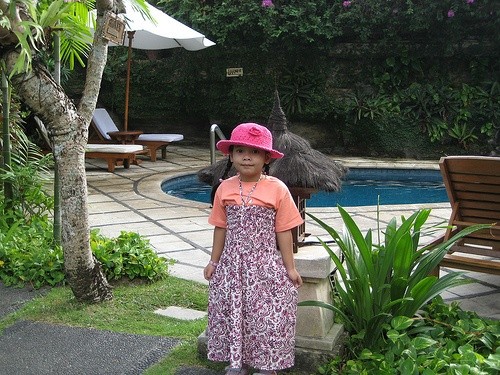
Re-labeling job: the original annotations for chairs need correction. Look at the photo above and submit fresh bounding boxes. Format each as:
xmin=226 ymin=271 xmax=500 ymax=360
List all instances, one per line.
xmin=418 ymin=156 xmax=500 ymax=279
xmin=34 ymin=115 xmax=143 ymax=171
xmin=91 ymin=109 xmax=184 ymax=159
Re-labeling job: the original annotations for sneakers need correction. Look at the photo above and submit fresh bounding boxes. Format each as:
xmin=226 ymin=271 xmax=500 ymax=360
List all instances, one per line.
xmin=224 ymin=366 xmax=248 ymax=375
xmin=253 ymin=370 xmax=277 ymax=375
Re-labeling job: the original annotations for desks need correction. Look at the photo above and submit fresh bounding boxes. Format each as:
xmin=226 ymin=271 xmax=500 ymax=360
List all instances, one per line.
xmin=105 ymin=131 xmax=145 ymax=165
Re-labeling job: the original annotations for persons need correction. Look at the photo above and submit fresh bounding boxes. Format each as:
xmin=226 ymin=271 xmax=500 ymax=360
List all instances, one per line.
xmin=204 ymin=123 xmax=304 ymax=375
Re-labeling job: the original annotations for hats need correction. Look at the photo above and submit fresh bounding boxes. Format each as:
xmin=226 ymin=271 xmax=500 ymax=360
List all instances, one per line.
xmin=216 ymin=122 xmax=284 ymax=158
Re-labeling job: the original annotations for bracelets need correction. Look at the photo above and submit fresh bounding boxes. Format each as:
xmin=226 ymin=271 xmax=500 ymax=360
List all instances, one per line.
xmin=210 ymin=260 xmax=218 ymax=266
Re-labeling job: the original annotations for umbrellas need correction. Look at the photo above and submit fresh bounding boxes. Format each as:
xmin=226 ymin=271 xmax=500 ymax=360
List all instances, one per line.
xmin=66 ymin=0 xmax=217 ymax=131
